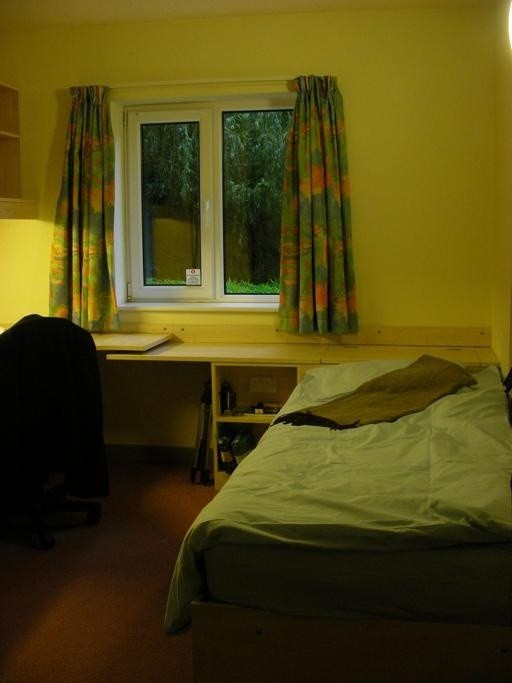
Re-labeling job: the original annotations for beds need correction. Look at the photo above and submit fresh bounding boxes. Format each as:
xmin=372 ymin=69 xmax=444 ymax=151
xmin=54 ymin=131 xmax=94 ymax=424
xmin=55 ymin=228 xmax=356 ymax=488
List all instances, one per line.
xmin=162 ymin=355 xmax=512 ymax=683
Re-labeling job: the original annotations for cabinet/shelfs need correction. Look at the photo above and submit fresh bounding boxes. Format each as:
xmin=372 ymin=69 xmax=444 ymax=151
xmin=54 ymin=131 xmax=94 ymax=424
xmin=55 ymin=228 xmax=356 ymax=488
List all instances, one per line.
xmin=0 ymin=82 xmax=41 ymax=219
xmin=210 ymin=362 xmax=301 ymax=493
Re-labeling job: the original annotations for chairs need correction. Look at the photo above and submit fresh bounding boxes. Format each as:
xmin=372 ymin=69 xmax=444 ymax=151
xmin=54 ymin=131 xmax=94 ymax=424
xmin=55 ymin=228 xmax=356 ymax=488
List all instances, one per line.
xmin=0 ymin=313 xmax=109 ymax=548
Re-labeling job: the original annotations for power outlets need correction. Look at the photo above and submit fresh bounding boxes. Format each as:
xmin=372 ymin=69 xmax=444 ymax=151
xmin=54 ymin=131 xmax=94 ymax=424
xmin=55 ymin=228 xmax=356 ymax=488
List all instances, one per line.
xmin=248 ymin=375 xmax=278 ymax=394
